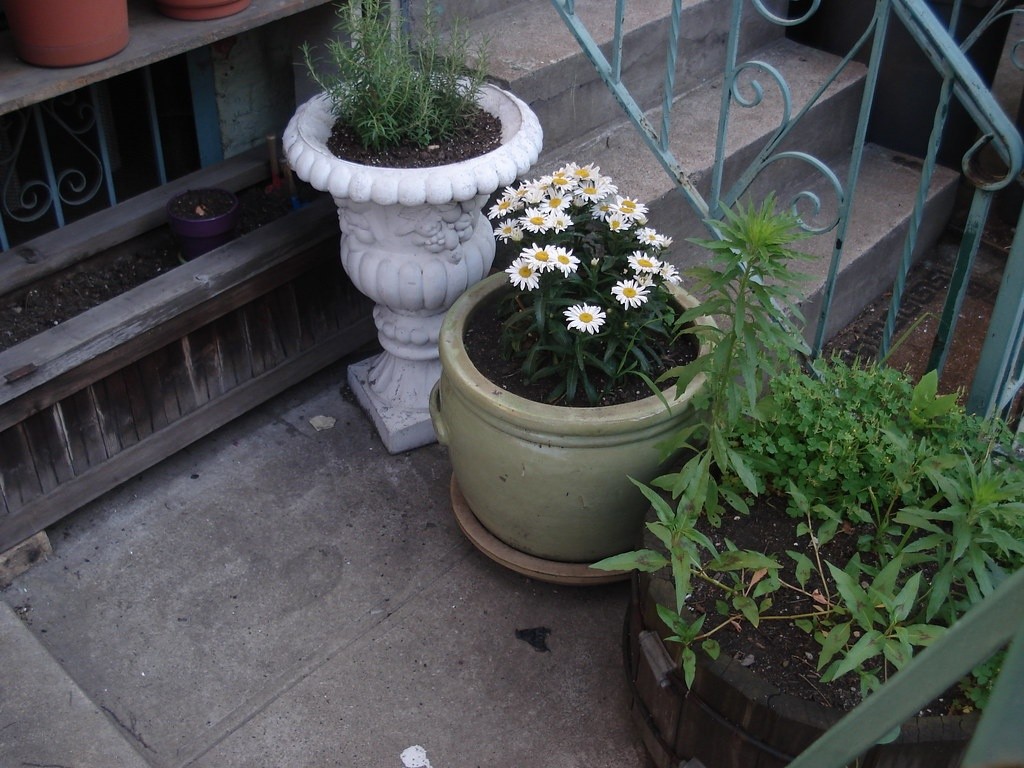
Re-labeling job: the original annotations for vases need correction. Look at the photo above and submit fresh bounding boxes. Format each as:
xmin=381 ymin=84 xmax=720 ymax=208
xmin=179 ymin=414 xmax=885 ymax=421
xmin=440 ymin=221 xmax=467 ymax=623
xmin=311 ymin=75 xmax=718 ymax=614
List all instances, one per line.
xmin=5 ymin=1 xmax=129 ymax=69
xmin=428 ymin=272 xmax=723 ymax=582
xmin=166 ymin=187 xmax=240 ymax=265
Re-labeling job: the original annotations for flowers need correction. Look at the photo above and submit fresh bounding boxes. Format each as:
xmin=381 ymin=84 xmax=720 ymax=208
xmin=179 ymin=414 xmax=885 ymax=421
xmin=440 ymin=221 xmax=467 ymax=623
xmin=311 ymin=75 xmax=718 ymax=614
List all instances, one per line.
xmin=484 ymin=162 xmax=676 ymax=404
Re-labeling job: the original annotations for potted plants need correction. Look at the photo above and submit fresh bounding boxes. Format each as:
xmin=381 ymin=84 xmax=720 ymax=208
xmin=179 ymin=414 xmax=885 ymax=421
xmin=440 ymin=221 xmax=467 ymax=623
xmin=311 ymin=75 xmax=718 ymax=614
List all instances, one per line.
xmin=622 ymin=191 xmax=1024 ymax=768
xmin=280 ymin=1 xmax=545 ymax=458
